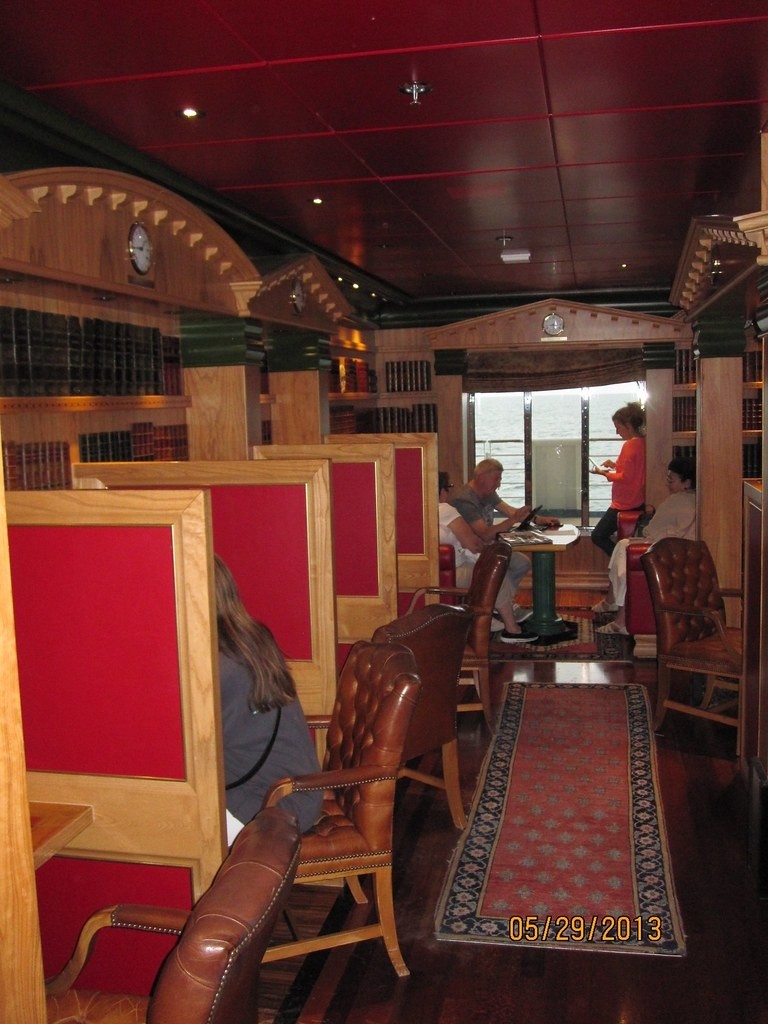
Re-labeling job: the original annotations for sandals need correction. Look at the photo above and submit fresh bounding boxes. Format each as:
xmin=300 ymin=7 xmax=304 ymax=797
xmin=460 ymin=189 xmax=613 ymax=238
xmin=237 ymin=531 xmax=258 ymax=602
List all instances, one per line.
xmin=590 ymin=599 xmax=618 ymax=612
xmin=594 ymin=621 xmax=630 ymax=636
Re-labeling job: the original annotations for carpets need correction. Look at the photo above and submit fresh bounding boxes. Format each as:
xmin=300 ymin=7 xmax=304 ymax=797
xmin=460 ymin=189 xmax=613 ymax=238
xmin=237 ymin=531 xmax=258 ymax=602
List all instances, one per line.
xmin=487 ymin=606 xmax=634 ymax=664
xmin=432 ymin=682 xmax=688 ymax=957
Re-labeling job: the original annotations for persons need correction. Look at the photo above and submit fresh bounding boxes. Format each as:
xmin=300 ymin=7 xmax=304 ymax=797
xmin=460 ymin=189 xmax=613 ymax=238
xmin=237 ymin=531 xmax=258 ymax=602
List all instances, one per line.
xmin=438 ymin=401 xmax=699 ymax=643
xmin=214 ymin=553 xmax=323 ymax=846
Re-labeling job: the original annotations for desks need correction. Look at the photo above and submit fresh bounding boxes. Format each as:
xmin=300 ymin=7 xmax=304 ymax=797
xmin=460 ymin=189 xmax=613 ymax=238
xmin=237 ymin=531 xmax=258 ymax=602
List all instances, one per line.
xmin=30 ymin=803 xmax=92 ymax=870
xmin=499 ymin=523 xmax=581 ymax=636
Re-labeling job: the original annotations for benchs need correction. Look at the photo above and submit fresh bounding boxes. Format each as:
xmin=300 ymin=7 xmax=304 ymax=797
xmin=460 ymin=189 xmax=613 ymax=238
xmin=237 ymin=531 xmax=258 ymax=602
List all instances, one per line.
xmin=617 ymin=509 xmax=658 ymax=660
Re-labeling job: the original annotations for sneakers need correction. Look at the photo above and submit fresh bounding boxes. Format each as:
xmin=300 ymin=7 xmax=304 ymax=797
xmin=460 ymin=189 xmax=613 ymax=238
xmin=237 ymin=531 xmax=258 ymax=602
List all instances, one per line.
xmin=492 ymin=603 xmax=533 ymax=623
xmin=500 ymin=626 xmax=539 ymax=644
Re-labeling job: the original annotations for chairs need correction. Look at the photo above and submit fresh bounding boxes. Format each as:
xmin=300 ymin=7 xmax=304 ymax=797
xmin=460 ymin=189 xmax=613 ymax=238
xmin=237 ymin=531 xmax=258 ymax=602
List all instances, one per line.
xmin=262 ymin=640 xmax=421 ymax=977
xmin=405 ymin=540 xmax=513 ymax=736
xmin=44 ymin=807 xmax=302 ymax=1024
xmin=640 ymin=536 xmax=741 ymax=759
xmin=371 ymin=603 xmax=475 ymax=830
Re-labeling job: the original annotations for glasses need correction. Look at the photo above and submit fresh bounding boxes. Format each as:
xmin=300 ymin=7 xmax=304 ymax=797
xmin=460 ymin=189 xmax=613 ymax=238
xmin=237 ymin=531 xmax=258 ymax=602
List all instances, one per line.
xmin=447 ymin=483 xmax=454 ymax=487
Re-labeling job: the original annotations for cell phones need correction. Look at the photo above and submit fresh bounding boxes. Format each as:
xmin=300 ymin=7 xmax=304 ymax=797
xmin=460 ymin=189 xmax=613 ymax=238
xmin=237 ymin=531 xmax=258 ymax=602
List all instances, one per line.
xmin=588 ymin=456 xmax=602 ymax=471
xmin=545 ymin=522 xmax=564 ymax=527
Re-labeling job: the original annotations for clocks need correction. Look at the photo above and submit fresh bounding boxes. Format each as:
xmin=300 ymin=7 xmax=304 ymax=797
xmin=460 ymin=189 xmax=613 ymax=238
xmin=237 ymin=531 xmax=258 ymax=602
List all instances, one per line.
xmin=542 ymin=313 xmax=565 ymax=336
xmin=290 ymin=277 xmax=304 ymax=313
xmin=128 ymin=222 xmax=153 ymax=275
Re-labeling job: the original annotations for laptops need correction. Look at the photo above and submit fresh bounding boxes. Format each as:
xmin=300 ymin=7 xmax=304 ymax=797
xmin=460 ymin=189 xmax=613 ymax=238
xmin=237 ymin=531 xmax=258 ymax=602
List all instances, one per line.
xmin=507 ymin=504 xmax=544 ymax=532
